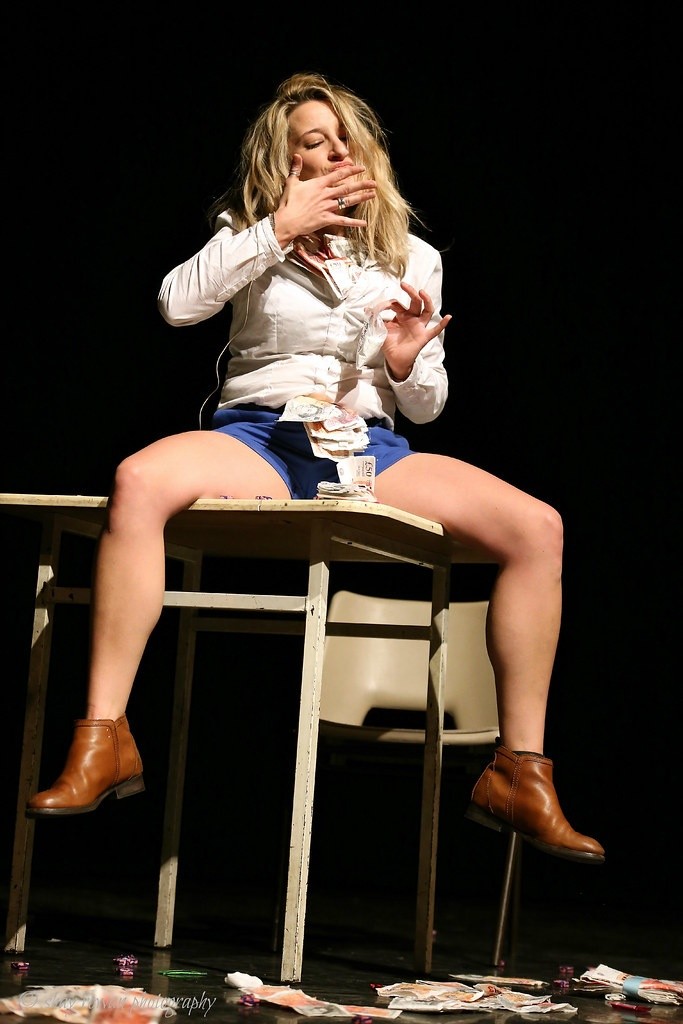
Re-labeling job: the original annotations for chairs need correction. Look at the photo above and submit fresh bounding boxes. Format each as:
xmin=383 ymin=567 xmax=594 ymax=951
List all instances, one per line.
xmin=272 ymin=591 xmax=529 ymax=975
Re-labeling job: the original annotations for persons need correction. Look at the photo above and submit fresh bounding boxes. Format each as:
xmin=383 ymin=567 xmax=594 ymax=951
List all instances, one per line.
xmin=26 ymin=76 xmax=606 ymax=863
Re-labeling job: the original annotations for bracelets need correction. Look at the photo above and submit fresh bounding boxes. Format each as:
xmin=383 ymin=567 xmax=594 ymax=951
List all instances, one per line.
xmin=269 ymin=211 xmax=276 ymax=235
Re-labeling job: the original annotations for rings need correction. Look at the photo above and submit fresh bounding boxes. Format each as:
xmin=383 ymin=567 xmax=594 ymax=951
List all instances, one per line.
xmin=337 ymin=198 xmax=346 ymax=210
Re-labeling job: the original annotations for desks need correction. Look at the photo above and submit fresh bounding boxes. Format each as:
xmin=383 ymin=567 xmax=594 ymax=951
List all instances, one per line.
xmin=0 ymin=494 xmax=456 ymax=984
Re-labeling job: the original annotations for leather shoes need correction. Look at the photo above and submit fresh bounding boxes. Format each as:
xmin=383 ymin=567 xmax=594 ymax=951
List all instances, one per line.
xmin=470 ymin=738 xmax=607 ymax=865
xmin=22 ymin=715 xmax=146 ymax=815
